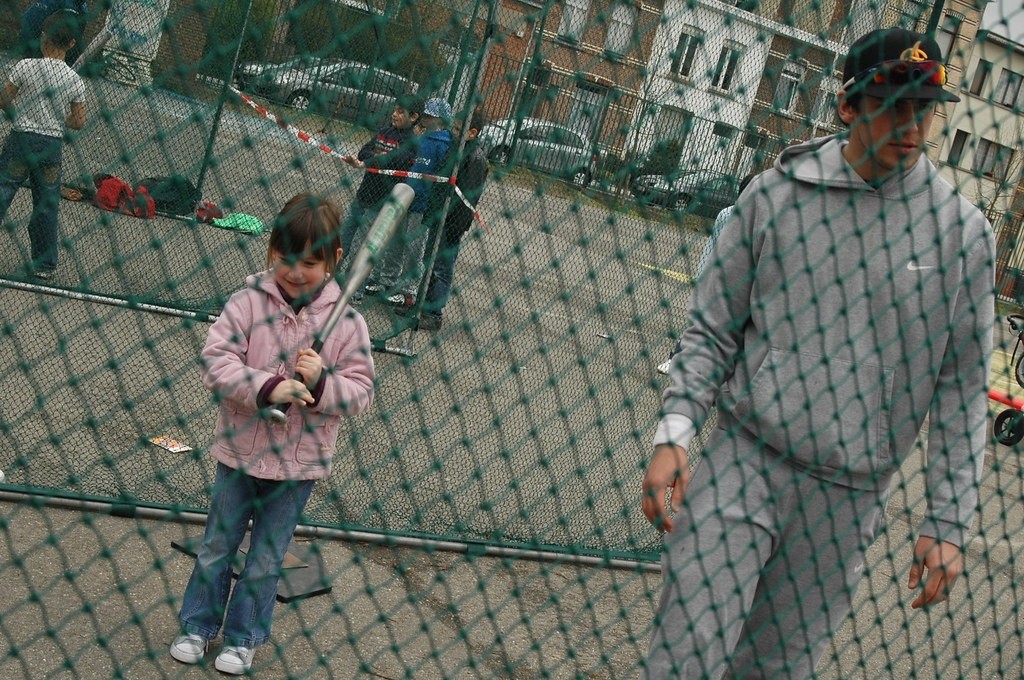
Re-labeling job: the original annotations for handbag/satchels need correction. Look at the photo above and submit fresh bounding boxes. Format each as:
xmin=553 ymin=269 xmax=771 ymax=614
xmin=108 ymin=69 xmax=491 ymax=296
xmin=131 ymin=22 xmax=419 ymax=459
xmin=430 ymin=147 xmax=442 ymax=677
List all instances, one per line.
xmin=92 ymin=173 xmax=155 ymax=218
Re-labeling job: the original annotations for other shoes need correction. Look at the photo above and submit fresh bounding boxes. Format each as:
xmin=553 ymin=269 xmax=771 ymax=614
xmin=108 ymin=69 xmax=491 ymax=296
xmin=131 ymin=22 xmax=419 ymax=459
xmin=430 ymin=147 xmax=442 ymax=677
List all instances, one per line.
xmin=405 ymin=294 xmax=417 ymax=304
xmin=33 ymin=267 xmax=56 ymax=278
xmin=365 ymin=281 xmax=392 ymax=295
xmin=394 ymin=305 xmax=416 ymax=316
xmin=419 ymin=313 xmax=442 ymax=330
xmin=384 ymin=292 xmax=407 ymax=305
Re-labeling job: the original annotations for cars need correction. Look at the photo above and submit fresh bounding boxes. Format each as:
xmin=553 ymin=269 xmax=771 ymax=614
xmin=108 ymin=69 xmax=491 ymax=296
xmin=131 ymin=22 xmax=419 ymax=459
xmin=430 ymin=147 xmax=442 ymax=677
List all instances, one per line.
xmin=476 ymin=116 xmax=598 ymax=188
xmin=629 ymin=169 xmax=742 ymax=218
xmin=236 ymin=55 xmax=322 ymax=87
xmin=251 ymin=58 xmax=418 ymax=132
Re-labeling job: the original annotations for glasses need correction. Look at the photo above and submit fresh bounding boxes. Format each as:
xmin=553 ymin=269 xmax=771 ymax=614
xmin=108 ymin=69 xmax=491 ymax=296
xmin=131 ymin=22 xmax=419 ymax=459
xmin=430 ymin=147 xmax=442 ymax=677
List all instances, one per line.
xmin=424 ymin=113 xmax=443 ymax=120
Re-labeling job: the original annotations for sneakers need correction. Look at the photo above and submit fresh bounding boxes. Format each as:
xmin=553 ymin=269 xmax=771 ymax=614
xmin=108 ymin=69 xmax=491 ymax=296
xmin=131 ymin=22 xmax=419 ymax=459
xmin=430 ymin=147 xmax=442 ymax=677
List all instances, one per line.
xmin=170 ymin=634 xmax=209 ymax=663
xmin=215 ymin=646 xmax=256 ymax=674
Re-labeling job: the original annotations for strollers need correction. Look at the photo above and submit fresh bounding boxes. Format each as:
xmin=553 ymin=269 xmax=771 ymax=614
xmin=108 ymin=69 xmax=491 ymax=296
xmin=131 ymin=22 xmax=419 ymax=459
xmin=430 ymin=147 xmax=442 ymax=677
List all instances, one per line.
xmin=994 ymin=313 xmax=1024 ymax=445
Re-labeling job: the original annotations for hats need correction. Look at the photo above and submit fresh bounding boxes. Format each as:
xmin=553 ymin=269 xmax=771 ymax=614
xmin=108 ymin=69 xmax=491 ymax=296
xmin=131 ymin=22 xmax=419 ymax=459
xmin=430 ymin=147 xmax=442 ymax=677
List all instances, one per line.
xmin=843 ymin=26 xmax=961 ymax=103
xmin=424 ymin=98 xmax=452 ymax=119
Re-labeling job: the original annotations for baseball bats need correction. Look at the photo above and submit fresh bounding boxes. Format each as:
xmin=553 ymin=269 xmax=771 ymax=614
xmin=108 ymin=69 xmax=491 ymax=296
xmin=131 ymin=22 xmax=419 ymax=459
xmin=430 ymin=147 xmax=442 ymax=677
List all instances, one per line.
xmin=269 ymin=182 xmax=415 ymax=423
xmin=70 ymin=26 xmax=114 ymax=73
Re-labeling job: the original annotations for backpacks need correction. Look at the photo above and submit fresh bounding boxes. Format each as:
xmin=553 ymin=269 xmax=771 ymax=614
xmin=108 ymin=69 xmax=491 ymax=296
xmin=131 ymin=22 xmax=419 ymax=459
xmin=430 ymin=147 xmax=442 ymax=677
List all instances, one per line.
xmin=134 ymin=176 xmax=196 ymax=215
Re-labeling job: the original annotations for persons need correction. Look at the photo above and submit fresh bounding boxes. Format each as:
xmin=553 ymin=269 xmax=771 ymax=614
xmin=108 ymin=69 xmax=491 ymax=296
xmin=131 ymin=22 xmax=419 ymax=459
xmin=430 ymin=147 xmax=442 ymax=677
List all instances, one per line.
xmin=20 ymin=0 xmax=90 ymax=62
xmin=335 ymin=93 xmax=490 ymax=332
xmin=166 ymin=193 xmax=376 ymax=679
xmin=640 ymin=26 xmax=1008 ymax=680
xmin=0 ymin=9 xmax=87 ymax=280
